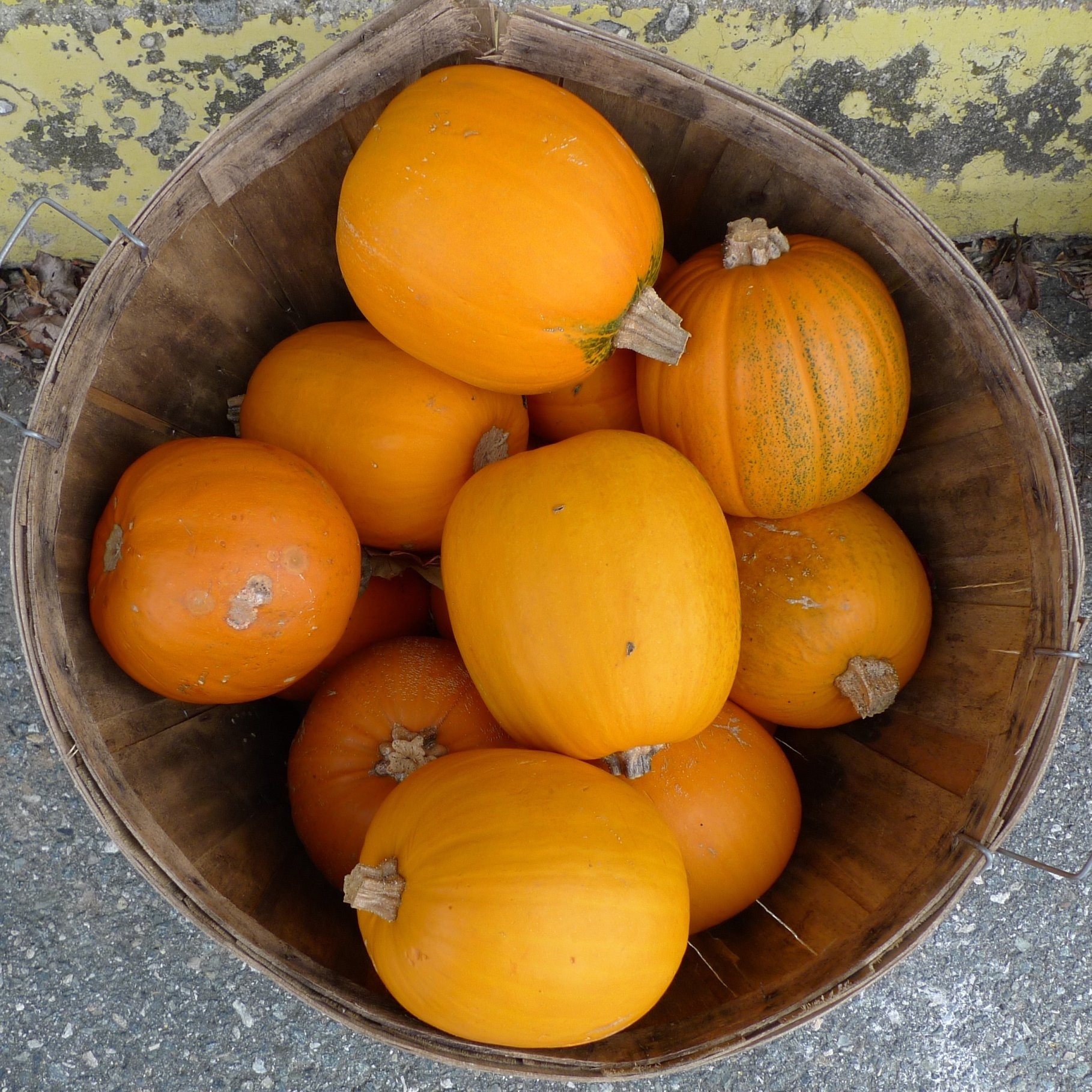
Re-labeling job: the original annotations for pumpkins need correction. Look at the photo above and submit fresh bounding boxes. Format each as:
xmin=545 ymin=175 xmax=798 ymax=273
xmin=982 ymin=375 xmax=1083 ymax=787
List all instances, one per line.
xmin=84 ymin=61 xmax=931 ymax=1052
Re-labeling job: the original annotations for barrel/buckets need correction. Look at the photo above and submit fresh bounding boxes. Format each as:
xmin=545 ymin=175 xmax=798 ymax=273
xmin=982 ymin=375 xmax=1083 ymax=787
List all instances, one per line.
xmin=1 ymin=1 xmax=1088 ymax=1084
